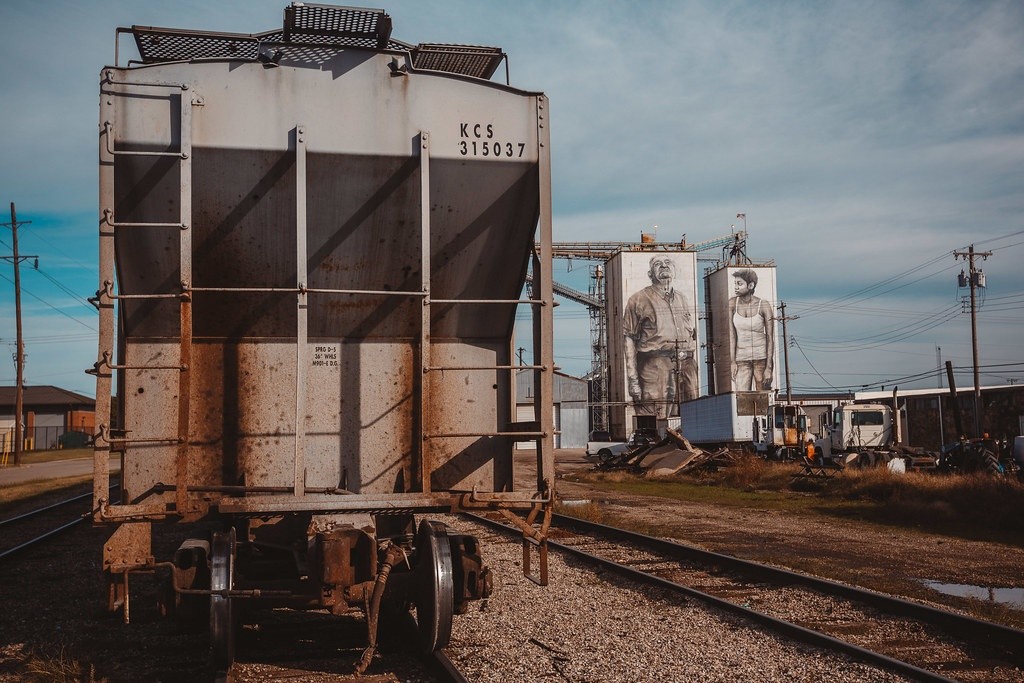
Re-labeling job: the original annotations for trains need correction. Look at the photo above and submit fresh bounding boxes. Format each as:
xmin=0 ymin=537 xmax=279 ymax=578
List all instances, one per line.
xmin=82 ymin=0 xmax=561 ymax=671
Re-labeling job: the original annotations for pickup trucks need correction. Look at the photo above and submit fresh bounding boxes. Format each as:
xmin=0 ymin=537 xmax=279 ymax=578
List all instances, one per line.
xmin=585 ymin=433 xmax=656 ymax=462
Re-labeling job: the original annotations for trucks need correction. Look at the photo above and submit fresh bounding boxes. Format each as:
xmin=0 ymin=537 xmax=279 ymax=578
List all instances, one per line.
xmin=814 ymin=385 xmax=939 ymax=473
